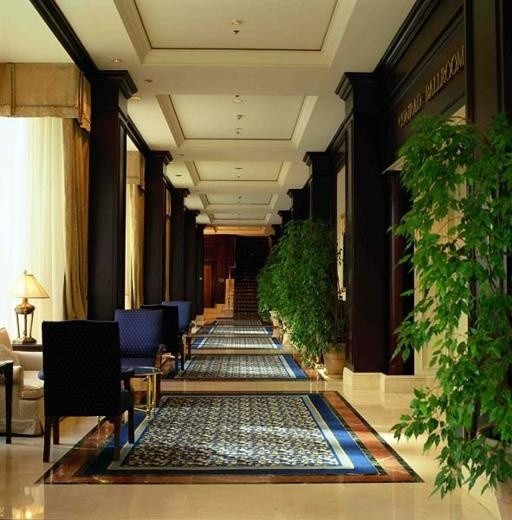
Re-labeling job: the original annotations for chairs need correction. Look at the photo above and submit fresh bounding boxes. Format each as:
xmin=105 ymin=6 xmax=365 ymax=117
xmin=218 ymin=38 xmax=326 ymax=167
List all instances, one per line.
xmin=0 ymin=327 xmax=61 ymax=435
xmin=41 ymin=300 xmax=192 ymax=461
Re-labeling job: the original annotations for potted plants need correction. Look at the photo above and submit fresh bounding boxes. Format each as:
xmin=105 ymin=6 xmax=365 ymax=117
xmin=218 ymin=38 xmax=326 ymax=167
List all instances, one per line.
xmin=394 ymin=115 xmax=512 ymax=520
xmin=258 ymin=216 xmax=349 ymax=377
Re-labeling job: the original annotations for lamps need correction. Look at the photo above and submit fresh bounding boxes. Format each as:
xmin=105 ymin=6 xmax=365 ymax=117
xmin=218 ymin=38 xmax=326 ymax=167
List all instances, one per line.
xmin=7 ymin=272 xmax=50 ymax=343
